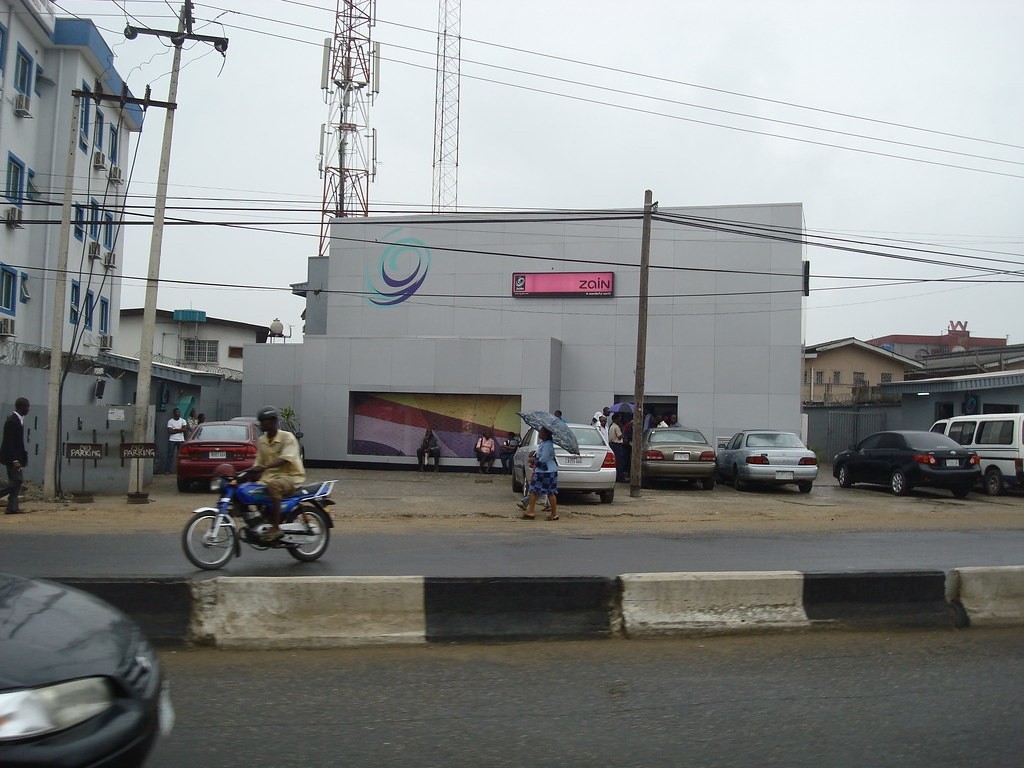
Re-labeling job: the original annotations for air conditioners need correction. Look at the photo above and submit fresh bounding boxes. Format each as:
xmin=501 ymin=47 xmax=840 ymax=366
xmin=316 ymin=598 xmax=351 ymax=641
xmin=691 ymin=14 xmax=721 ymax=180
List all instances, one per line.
xmin=8 ymin=206 xmax=23 ymax=229
xmin=99 ymin=334 xmax=113 ymax=350
xmin=110 ymin=166 xmax=121 ymax=182
xmin=0 ymin=317 xmax=16 ymax=337
xmin=88 ymin=242 xmax=101 ymax=259
xmin=94 ymin=151 xmax=106 ymax=170
xmin=16 ymin=93 xmax=31 ymax=117
xmin=103 ymin=252 xmax=116 ymax=268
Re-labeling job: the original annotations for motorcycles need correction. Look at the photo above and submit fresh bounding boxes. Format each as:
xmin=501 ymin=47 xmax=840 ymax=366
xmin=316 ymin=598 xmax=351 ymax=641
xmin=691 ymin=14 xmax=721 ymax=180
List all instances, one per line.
xmin=181 ymin=462 xmax=341 ymax=571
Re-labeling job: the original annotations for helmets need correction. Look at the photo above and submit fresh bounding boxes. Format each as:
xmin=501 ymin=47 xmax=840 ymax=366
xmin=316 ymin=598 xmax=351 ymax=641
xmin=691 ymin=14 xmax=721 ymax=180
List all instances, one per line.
xmin=211 ymin=464 xmax=234 ymax=482
xmin=258 ymin=405 xmax=278 ymax=421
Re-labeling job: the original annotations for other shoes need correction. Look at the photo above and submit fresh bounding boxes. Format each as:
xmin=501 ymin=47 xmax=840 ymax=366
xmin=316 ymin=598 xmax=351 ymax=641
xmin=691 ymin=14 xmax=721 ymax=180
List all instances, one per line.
xmin=517 ymin=503 xmax=527 ymax=510
xmin=434 ymin=469 xmax=438 ymax=472
xmin=541 ymin=508 xmax=552 ymax=511
xmin=262 ymin=530 xmax=284 ymax=541
xmin=618 ymin=478 xmax=630 ymax=483
xmin=166 ymin=471 xmax=170 ymax=475
xmin=4 ymin=508 xmax=23 ymax=514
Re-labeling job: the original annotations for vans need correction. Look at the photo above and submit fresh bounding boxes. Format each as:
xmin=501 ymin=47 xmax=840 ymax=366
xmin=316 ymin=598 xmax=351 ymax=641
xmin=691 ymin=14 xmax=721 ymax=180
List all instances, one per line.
xmin=929 ymin=413 xmax=1024 ymax=497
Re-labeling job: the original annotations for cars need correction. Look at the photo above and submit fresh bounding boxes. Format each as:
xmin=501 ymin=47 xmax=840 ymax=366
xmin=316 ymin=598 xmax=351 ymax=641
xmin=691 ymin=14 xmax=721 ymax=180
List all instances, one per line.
xmin=176 ymin=419 xmax=266 ymax=494
xmin=509 ymin=422 xmax=617 ymax=504
xmin=230 ymin=416 xmax=305 ymax=466
xmin=719 ymin=429 xmax=818 ymax=493
xmin=629 ymin=426 xmax=718 ymax=491
xmin=0 ymin=570 xmax=177 ymax=768
xmin=832 ymin=429 xmax=982 ymax=499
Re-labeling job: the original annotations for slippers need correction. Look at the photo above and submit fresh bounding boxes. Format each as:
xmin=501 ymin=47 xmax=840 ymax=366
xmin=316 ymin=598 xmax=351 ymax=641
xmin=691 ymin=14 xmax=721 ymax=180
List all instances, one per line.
xmin=545 ymin=514 xmax=559 ymax=521
xmin=522 ymin=514 xmax=535 ymax=520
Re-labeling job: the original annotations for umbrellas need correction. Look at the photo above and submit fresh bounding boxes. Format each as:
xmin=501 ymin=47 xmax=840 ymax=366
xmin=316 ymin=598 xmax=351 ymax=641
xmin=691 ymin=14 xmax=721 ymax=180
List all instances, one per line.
xmin=515 ymin=411 xmax=580 ymax=458
xmin=607 ymin=402 xmax=634 ymax=418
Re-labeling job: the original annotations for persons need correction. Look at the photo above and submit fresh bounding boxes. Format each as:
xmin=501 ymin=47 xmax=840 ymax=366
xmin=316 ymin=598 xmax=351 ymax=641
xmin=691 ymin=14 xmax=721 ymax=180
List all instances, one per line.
xmin=475 ymin=431 xmax=496 ymax=474
xmin=500 ymin=432 xmax=519 ymax=475
xmin=185 ymin=408 xmax=199 ymax=441
xmin=0 ymin=397 xmax=30 ymax=514
xmin=591 ymin=407 xmax=681 ymax=483
xmin=416 ymin=427 xmax=441 ymax=472
xmin=516 ymin=427 xmax=559 ymax=521
xmin=555 ymin=410 xmax=568 ymax=423
xmin=235 ymin=405 xmax=307 ymax=542
xmin=163 ymin=408 xmax=188 ymax=474
xmin=198 ymin=414 xmax=205 ymax=424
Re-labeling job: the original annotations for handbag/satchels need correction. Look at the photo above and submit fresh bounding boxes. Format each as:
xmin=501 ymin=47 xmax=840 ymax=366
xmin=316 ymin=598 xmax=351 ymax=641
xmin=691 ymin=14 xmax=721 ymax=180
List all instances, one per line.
xmin=425 ymin=453 xmax=428 ymax=465
xmin=474 ymin=437 xmax=483 ymax=453
